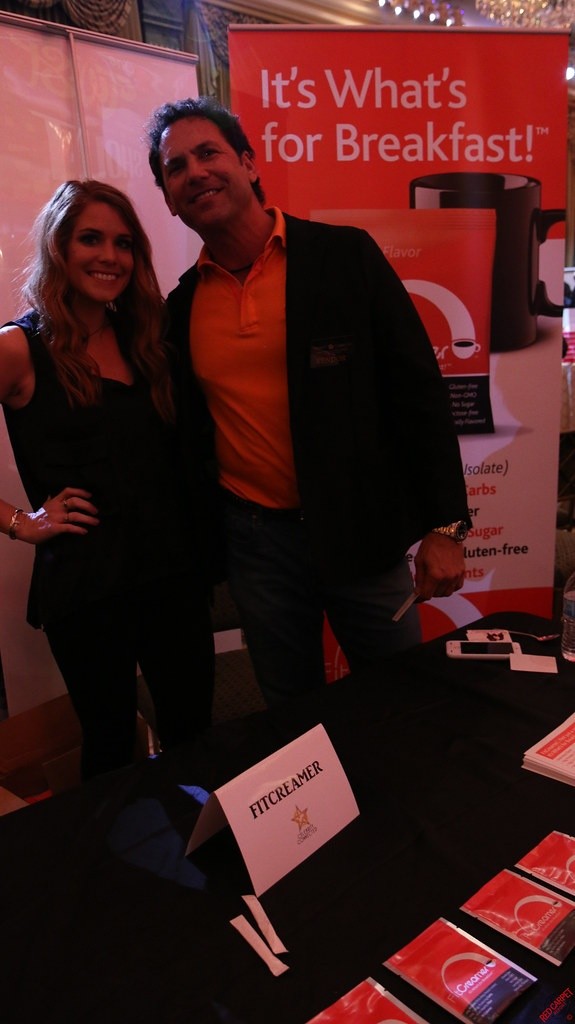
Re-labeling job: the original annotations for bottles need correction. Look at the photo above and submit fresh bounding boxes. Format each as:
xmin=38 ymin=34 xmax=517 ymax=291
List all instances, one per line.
xmin=561 ymin=572 xmax=575 ymax=661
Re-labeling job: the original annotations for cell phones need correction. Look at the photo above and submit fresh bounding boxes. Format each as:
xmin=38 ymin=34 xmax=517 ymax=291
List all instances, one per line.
xmin=445 ymin=641 xmax=522 ymax=659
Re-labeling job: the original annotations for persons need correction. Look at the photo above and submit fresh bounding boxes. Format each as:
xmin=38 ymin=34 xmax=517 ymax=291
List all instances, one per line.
xmin=148 ymin=99 xmax=472 ymax=710
xmin=0 ymin=181 xmax=224 ymax=782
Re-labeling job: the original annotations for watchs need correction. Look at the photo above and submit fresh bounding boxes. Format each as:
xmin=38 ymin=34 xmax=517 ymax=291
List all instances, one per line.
xmin=432 ymin=521 xmax=469 ymax=543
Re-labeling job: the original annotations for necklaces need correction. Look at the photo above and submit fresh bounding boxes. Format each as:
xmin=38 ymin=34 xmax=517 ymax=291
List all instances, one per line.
xmin=228 ymin=262 xmax=253 ymax=273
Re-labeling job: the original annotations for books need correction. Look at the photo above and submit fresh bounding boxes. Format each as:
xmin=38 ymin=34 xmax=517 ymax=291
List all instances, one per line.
xmin=520 ymin=711 xmax=575 ymax=787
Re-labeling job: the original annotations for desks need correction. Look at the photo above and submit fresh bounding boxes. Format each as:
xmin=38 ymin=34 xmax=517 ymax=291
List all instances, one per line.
xmin=0 ymin=610 xmax=575 ymax=1024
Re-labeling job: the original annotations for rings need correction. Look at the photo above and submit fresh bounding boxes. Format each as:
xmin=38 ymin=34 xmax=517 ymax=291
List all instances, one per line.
xmin=63 ymin=499 xmax=68 ymax=508
xmin=64 ymin=512 xmax=69 ymax=522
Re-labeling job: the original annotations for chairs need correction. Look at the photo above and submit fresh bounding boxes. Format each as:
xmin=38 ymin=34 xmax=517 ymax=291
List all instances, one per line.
xmin=130 ymin=581 xmax=268 ymax=738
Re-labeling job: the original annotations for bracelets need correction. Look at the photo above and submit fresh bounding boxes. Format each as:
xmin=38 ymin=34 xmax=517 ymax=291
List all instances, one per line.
xmin=9 ymin=508 xmax=24 ymax=540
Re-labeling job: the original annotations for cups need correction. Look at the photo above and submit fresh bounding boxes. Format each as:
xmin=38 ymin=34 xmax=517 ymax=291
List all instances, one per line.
xmin=452 ymin=340 xmax=480 ymax=359
xmin=410 ymin=173 xmax=566 ymax=352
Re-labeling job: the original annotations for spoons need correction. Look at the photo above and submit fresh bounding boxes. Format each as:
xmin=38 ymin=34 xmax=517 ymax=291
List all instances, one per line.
xmin=493 ymin=629 xmax=559 ymax=640
